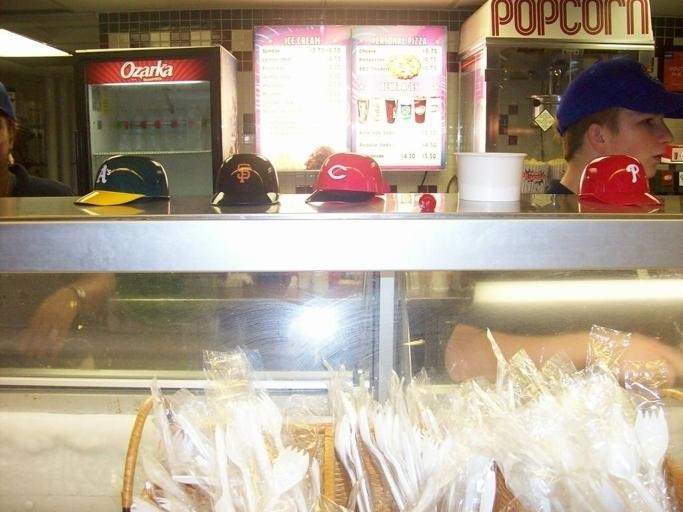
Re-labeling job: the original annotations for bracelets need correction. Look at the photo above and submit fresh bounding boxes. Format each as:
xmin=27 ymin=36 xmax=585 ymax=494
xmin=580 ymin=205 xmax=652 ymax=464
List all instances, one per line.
xmin=68 ymin=283 xmax=90 ymax=308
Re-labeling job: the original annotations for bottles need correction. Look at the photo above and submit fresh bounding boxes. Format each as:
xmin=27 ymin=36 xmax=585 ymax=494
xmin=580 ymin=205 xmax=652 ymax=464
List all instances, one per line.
xmin=356 ymin=95 xmax=441 ymax=124
xmin=115 ymin=102 xmax=212 ymax=152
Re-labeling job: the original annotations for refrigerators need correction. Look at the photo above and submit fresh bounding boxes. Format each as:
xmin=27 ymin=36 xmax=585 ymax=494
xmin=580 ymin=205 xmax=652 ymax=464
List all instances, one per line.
xmin=74 ymin=45 xmax=244 ymax=198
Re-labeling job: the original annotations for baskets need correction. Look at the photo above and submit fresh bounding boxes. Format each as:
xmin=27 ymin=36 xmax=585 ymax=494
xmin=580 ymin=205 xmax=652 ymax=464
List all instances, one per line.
xmin=122 ymin=387 xmax=682 ymax=512
xmin=120 ymin=387 xmax=682 ymax=512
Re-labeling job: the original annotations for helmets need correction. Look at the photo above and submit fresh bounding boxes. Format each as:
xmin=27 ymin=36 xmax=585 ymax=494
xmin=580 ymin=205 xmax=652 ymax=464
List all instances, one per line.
xmin=210 ymin=154 xmax=280 ymax=207
xmin=579 ymin=154 xmax=663 ymax=206
xmin=305 ymin=153 xmax=384 ymax=204
xmin=73 ymin=153 xmax=172 ymax=205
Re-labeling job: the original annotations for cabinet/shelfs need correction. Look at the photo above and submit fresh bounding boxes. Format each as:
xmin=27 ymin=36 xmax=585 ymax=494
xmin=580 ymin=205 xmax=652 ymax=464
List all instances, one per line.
xmin=0 ymin=192 xmax=683 ymax=512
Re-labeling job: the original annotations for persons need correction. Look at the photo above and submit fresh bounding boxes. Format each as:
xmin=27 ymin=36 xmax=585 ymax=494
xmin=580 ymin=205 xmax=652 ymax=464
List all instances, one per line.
xmin=0 ymin=78 xmax=118 ymax=366
xmin=422 ymin=54 xmax=683 ymax=392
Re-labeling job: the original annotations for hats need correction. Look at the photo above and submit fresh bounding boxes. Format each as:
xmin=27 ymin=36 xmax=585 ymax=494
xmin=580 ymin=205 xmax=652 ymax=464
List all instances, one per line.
xmin=556 ymin=60 xmax=683 ymax=134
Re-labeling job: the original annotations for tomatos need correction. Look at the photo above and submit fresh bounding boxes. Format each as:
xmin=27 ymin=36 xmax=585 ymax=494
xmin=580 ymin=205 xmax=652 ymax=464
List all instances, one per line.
xmin=419 ymin=194 xmax=436 ymax=212
